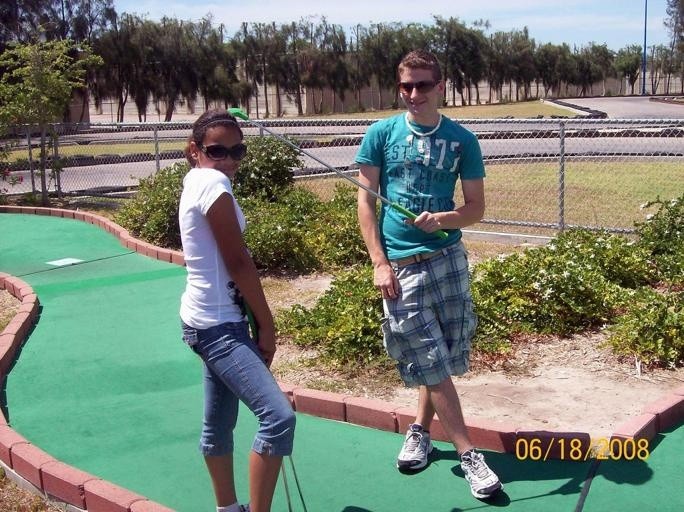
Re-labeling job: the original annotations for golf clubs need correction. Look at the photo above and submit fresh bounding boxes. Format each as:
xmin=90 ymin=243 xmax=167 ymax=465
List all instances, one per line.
xmin=242 ymin=304 xmax=307 ymax=512
xmin=227 ymin=107 xmax=448 ymax=240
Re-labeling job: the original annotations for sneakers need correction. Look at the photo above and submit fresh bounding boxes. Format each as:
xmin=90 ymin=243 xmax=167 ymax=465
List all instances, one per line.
xmin=460 ymin=449 xmax=504 ymax=499
xmin=397 ymin=423 xmax=433 ymax=469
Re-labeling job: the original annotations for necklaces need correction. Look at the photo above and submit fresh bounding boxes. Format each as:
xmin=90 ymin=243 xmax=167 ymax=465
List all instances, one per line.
xmin=403 ymin=109 xmax=442 ymax=139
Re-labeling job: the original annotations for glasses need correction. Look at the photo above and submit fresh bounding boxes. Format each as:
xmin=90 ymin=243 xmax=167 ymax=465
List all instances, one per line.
xmin=197 ymin=143 xmax=248 ymax=161
xmin=398 ymin=80 xmax=440 ymax=93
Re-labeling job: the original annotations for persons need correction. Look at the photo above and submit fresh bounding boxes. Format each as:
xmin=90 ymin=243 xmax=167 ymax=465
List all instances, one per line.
xmin=354 ymin=51 xmax=504 ymax=502
xmin=177 ymin=107 xmax=297 ymax=512
